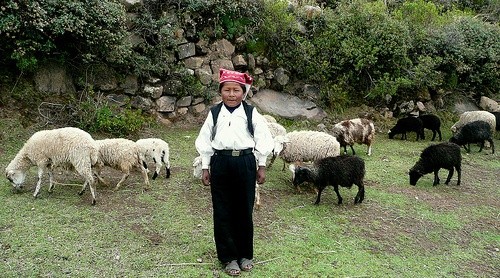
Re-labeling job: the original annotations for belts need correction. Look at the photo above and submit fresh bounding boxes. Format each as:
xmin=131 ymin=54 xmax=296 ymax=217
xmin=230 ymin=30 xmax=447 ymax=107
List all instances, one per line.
xmin=217 ymin=149 xmax=254 ymax=157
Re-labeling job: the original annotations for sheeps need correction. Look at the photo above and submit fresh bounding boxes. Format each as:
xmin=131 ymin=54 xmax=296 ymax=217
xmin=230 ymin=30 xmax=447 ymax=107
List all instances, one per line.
xmin=409 ymin=141 xmax=462 ymax=187
xmin=95 ymin=138 xmax=150 ymax=191
xmin=449 ymin=120 xmax=495 ymax=154
xmin=136 ymin=138 xmax=170 ymax=180
xmin=273 ymin=130 xmax=340 ymax=163
xmin=252 ymin=181 xmax=260 ymax=212
xmin=388 ymin=114 xmax=442 ymax=141
xmin=293 ymin=153 xmax=365 ymax=206
xmin=192 ymin=156 xmax=211 ymax=186
xmin=4 ymin=127 xmax=98 ymax=205
xmin=266 ymin=123 xmax=287 ymax=171
xmin=331 ymin=118 xmax=375 ymax=156
xmin=262 ymin=115 xmax=277 ymax=124
xmin=451 ymin=111 xmax=496 ymax=137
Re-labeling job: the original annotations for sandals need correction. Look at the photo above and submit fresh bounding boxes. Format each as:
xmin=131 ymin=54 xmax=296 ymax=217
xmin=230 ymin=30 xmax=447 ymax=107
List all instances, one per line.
xmin=225 ymin=260 xmax=242 ymax=276
xmin=240 ymin=257 xmax=255 ymax=272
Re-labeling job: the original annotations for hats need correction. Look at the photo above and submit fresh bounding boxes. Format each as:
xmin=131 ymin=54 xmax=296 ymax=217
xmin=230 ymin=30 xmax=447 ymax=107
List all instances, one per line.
xmin=218 ymin=67 xmax=254 ymax=102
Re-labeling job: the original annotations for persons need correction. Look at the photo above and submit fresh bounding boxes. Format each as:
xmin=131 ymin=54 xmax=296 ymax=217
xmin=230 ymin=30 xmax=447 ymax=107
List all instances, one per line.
xmin=195 ymin=69 xmax=274 ymax=275
